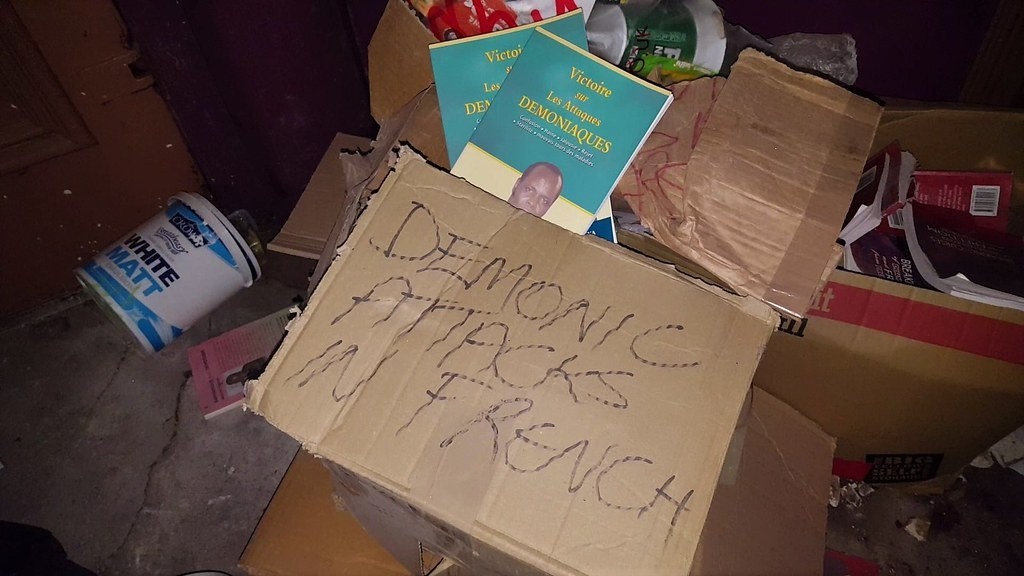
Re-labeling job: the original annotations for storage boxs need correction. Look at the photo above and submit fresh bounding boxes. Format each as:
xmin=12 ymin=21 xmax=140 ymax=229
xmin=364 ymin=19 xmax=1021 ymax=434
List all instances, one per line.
xmin=233 ymin=0 xmax=1024 ymax=576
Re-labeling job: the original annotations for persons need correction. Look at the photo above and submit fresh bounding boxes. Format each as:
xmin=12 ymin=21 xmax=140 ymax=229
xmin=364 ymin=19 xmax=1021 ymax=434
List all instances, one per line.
xmin=507 ymin=161 xmax=564 ymax=218
xmin=226 ymin=356 xmax=267 ymax=386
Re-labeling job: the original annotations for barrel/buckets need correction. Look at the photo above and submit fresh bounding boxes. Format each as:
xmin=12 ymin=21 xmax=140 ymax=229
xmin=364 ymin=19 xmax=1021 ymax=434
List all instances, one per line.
xmin=72 ymin=190 xmax=262 ymax=359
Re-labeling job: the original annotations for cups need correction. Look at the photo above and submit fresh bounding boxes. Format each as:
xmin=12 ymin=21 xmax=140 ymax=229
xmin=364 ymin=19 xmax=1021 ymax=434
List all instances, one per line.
xmin=592 ymin=0 xmax=729 ymax=84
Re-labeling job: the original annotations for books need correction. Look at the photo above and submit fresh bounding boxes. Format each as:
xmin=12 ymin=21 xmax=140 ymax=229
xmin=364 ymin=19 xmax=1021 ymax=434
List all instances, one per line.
xmin=839 ymin=140 xmax=1024 ymax=312
xmin=186 ymin=302 xmax=303 ymax=420
xmin=429 ymin=8 xmax=673 ymax=243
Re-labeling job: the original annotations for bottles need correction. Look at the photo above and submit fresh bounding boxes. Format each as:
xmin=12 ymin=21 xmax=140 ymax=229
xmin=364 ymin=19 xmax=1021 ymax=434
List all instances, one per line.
xmin=225 ymin=208 xmax=265 ymax=254
